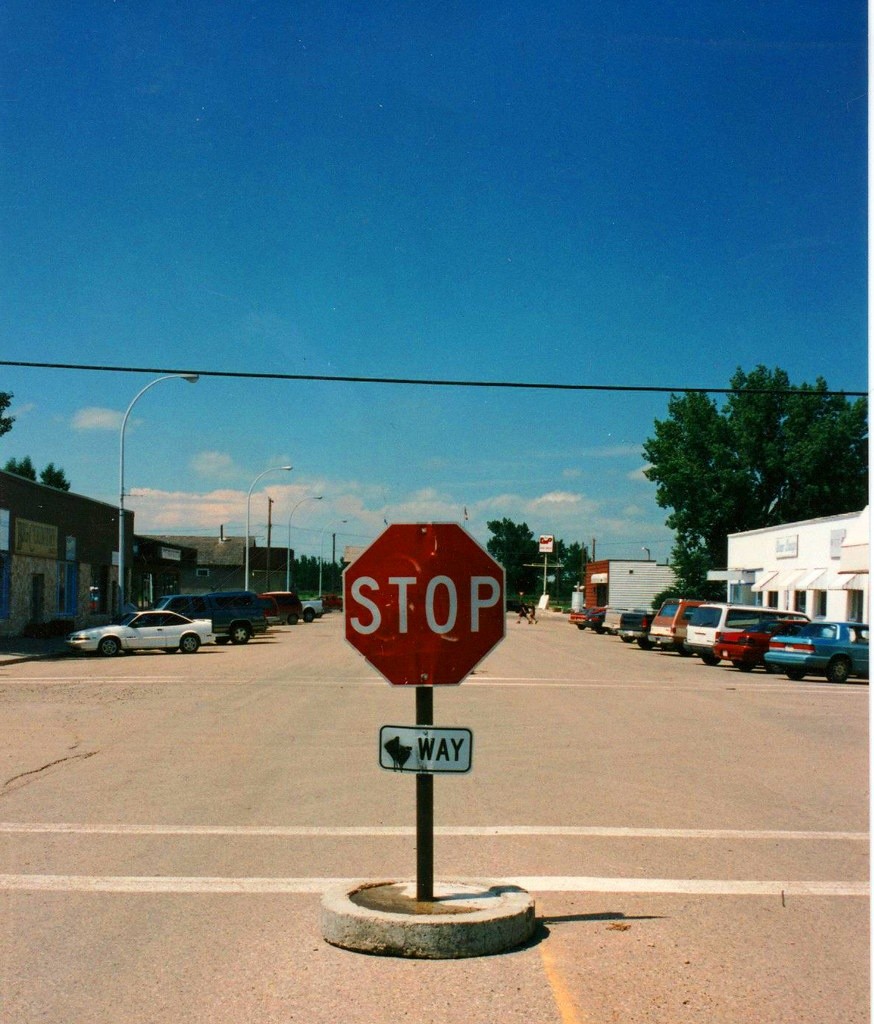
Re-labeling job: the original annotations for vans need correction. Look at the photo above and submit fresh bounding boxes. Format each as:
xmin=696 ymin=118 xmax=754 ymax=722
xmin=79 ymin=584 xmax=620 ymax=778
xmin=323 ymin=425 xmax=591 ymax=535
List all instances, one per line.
xmin=682 ymin=604 xmax=814 ymax=666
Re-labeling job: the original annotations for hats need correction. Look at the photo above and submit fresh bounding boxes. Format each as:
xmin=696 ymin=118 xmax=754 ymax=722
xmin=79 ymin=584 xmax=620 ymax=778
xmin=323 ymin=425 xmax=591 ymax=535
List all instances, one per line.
xmin=87 ymin=586 xmax=98 ymax=592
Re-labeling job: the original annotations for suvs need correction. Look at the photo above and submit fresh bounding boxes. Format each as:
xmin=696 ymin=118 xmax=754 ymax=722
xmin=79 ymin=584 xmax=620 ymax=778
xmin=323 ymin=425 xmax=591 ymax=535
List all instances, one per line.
xmin=258 ymin=591 xmax=305 ymax=626
xmin=647 ymin=599 xmax=717 ymax=657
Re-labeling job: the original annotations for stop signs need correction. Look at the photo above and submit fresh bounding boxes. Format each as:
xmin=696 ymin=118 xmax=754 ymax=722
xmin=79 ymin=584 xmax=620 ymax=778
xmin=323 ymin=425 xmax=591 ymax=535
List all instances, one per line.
xmin=345 ymin=522 xmax=508 ymax=688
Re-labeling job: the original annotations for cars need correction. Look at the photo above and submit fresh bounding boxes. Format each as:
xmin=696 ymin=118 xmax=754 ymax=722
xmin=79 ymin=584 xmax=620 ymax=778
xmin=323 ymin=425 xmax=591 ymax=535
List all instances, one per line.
xmin=568 ymin=607 xmax=628 ymax=634
xmin=64 ymin=609 xmax=218 ymax=657
xmin=714 ymin=622 xmax=814 ymax=672
xmin=763 ymin=620 xmax=868 ymax=685
xmin=319 ymin=595 xmax=344 ymax=614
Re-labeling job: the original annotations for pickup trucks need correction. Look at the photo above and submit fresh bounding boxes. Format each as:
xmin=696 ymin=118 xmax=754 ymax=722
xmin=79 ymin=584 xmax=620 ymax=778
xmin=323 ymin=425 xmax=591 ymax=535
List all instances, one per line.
xmin=148 ymin=589 xmax=269 ymax=644
xmin=603 ymin=605 xmax=658 ymax=650
xmin=301 ymin=600 xmax=324 ymax=623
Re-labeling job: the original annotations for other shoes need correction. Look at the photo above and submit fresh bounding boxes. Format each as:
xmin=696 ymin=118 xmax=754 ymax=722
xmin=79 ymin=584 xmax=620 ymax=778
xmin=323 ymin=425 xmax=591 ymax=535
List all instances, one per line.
xmin=529 ymin=621 xmax=532 ymax=624
xmin=535 ymin=620 xmax=538 ymax=624
xmin=517 ymin=621 xmax=521 ymax=624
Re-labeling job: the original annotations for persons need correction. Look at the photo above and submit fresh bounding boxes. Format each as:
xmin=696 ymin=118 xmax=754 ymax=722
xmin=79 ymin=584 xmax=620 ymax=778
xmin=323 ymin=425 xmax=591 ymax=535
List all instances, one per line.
xmin=515 ymin=599 xmax=539 ymax=624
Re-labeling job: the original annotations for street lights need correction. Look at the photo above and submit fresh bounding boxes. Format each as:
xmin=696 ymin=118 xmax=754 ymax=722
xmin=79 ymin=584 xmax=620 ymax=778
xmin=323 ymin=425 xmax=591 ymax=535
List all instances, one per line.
xmin=317 ymin=519 xmax=348 ymax=599
xmin=119 ymin=373 xmax=201 ymax=620
xmin=642 ymin=546 xmax=651 ymax=559
xmin=286 ymin=496 xmax=322 ymax=593
xmin=244 ymin=465 xmax=292 ymax=592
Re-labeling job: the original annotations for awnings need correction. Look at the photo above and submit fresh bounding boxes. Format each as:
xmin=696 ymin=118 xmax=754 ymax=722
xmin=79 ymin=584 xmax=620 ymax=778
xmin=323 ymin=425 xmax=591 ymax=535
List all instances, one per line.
xmin=750 ymin=568 xmax=870 ymax=592
